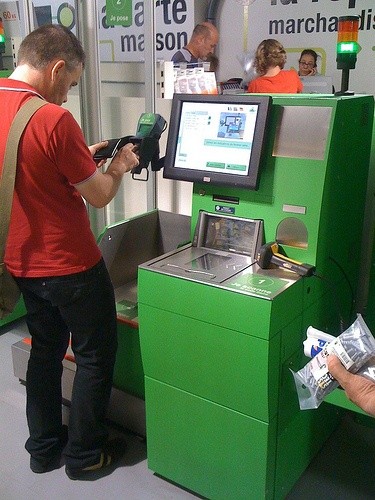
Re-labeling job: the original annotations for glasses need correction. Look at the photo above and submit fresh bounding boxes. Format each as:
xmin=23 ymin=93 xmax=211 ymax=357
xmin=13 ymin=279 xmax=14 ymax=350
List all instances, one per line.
xmin=299 ymin=62 xmax=314 ymax=66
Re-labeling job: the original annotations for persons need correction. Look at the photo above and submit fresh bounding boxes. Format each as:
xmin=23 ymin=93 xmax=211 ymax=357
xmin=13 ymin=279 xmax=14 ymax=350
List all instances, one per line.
xmin=296 ymin=49 xmax=318 ymax=76
xmin=0 ymin=24 xmax=140 ymax=480
xmin=170 ymin=22 xmax=219 ymax=69
xmin=246 ymin=39 xmax=303 ymax=94
xmin=327 ymin=354 xmax=375 ymax=416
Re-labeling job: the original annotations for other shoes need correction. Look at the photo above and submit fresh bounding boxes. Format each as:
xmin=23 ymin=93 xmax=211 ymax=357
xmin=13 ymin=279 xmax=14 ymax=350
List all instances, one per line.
xmin=65 ymin=437 xmax=126 ymax=481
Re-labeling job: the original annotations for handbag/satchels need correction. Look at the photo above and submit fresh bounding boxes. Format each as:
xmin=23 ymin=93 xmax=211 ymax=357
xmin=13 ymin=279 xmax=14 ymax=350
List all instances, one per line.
xmin=0 ymin=263 xmax=22 ymax=321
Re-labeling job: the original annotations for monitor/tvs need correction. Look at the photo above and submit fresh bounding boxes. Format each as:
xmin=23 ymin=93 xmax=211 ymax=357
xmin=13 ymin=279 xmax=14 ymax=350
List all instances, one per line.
xmin=299 ymin=76 xmax=333 ymax=95
xmin=162 ymin=93 xmax=273 ymax=189
